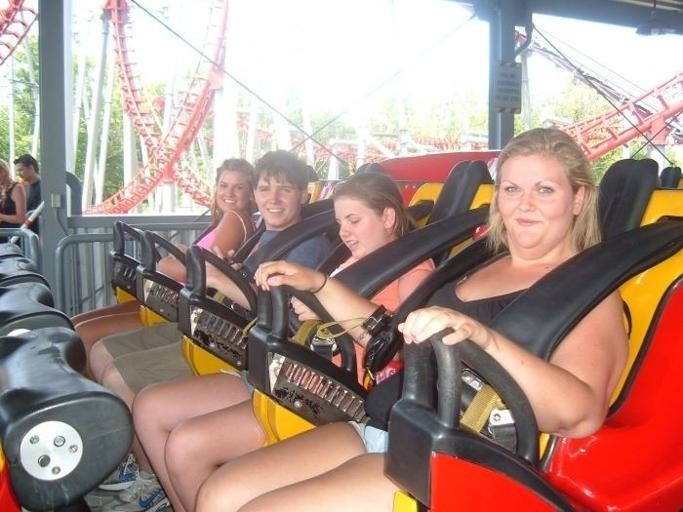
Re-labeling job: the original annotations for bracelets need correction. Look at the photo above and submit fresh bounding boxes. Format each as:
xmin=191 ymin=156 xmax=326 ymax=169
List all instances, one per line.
xmin=311 ymin=271 xmax=327 ymax=294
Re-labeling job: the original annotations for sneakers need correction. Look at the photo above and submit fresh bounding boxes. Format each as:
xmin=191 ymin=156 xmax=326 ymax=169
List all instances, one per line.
xmin=102 ymin=472 xmax=171 ymax=512
xmin=99 ymin=454 xmax=141 ymax=491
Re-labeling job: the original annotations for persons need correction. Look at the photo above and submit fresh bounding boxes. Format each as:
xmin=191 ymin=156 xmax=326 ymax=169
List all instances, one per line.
xmin=89 ymin=150 xmax=331 ymax=512
xmin=193 ymin=128 xmax=629 ymax=512
xmin=0 ymin=159 xmax=26 ymax=245
xmin=132 ymin=171 xmax=435 ymax=512
xmin=70 ymin=158 xmax=256 ymax=381
xmin=13 ymin=153 xmax=41 ymax=235
